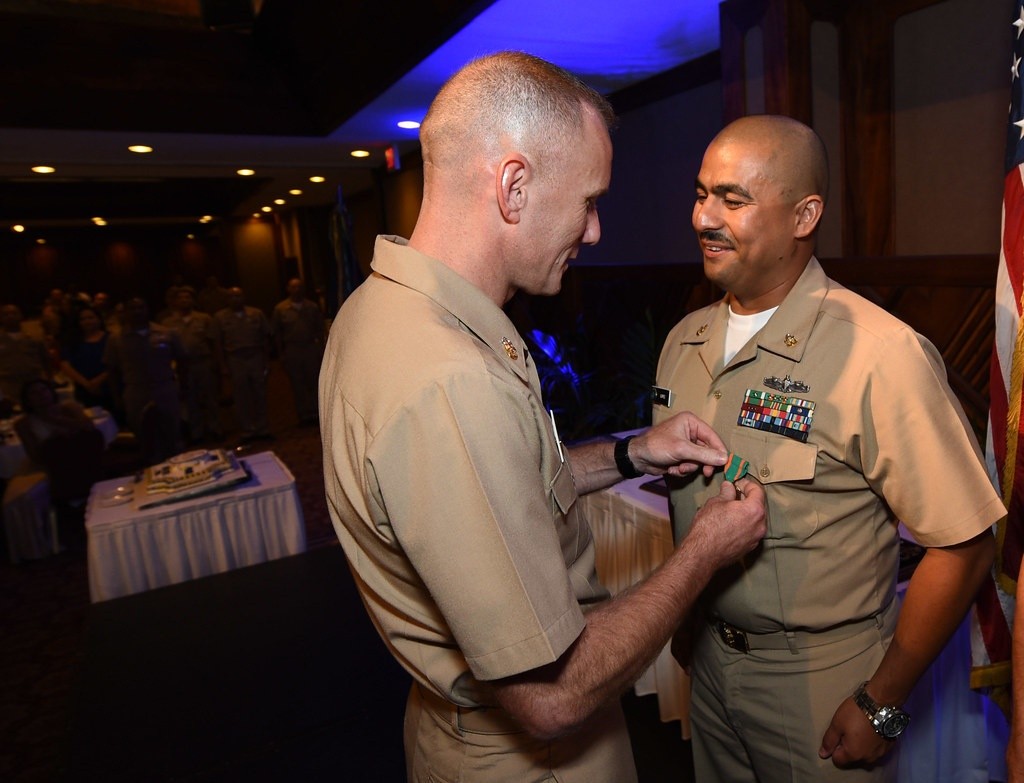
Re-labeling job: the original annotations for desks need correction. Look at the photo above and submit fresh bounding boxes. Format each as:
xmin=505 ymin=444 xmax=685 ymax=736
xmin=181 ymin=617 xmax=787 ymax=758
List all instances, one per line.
xmin=0 ymin=406 xmax=119 ymax=480
xmin=567 ymin=425 xmax=991 ymax=739
xmin=85 ymin=449 xmax=307 ymax=604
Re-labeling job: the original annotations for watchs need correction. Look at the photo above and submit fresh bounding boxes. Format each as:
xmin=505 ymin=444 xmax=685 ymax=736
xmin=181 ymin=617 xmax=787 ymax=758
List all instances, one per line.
xmin=854 ymin=681 xmax=912 ymax=741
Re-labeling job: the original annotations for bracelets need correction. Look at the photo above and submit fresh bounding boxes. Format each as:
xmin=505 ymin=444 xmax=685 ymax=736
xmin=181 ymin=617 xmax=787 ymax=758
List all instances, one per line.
xmin=614 ymin=435 xmax=646 ymax=479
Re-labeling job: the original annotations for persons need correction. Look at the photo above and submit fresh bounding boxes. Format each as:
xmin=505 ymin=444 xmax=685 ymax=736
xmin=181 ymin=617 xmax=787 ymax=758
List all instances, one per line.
xmin=0 ymin=284 xmax=183 ymax=553
xmin=317 ymin=50 xmax=769 ymax=783
xmin=266 ymin=277 xmax=325 ymax=429
xmin=212 ymin=282 xmax=277 ymax=442
xmin=160 ymin=285 xmax=229 ymax=451
xmin=650 ymin=112 xmax=1010 ymax=783
xmin=199 ymin=272 xmax=228 ymax=316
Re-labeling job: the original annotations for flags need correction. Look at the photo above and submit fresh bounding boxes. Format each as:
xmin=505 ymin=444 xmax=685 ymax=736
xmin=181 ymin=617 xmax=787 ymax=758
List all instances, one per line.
xmin=965 ymin=0 xmax=1024 ymax=727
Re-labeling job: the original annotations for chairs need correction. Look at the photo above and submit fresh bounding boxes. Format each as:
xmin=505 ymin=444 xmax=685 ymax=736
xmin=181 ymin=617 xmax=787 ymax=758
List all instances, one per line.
xmin=3 ymin=472 xmax=62 ymax=563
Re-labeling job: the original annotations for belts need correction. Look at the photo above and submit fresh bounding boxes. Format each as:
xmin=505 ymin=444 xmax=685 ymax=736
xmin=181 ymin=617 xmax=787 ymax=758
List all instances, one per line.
xmin=417 ymin=681 xmax=524 ymax=734
xmin=712 ymin=602 xmax=891 ymax=655
